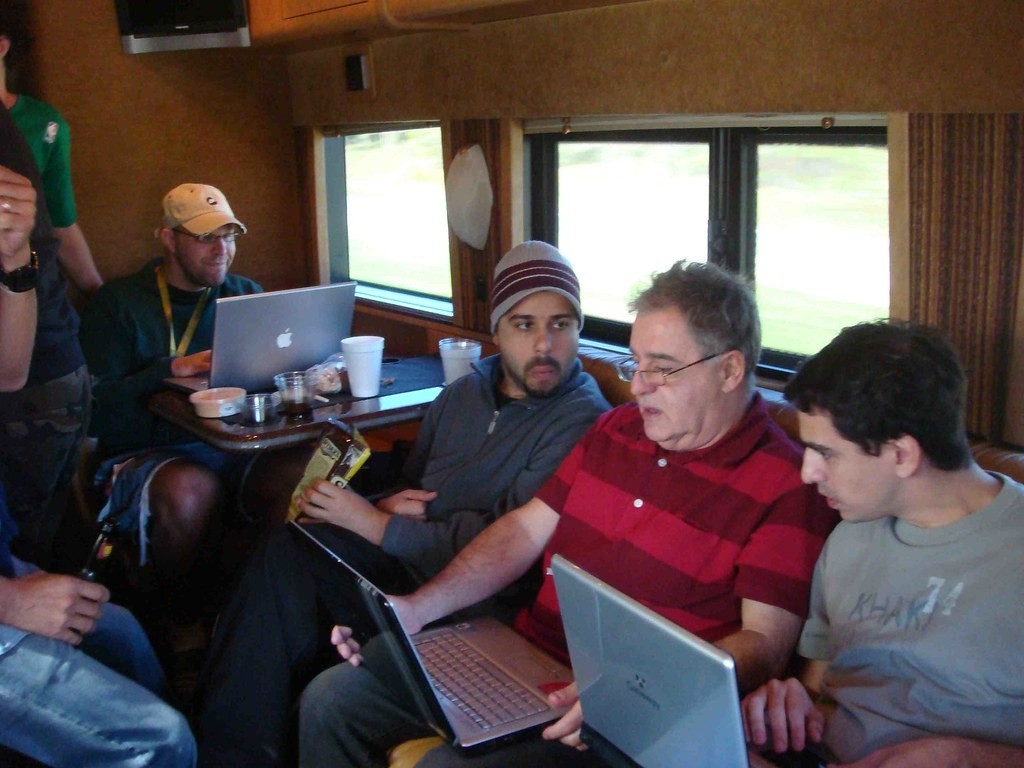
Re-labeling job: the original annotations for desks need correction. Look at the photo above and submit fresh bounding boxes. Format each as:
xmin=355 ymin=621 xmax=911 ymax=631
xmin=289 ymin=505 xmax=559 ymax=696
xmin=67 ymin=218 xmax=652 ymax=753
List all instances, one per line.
xmin=146 ymin=352 xmax=445 ymax=527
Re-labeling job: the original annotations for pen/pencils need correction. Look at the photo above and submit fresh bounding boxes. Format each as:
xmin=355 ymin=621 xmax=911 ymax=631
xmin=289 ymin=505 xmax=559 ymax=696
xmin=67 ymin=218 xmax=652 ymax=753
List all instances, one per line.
xmin=315 ymin=395 xmax=329 ymax=403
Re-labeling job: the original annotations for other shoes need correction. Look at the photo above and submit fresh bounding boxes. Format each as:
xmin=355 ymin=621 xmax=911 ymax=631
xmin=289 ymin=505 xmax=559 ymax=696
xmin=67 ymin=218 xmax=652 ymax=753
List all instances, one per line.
xmin=170 ymin=622 xmax=209 ymax=694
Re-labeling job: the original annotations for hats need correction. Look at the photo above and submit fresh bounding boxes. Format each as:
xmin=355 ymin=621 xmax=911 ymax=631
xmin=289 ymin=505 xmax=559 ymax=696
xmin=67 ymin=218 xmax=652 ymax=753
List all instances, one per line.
xmin=154 ymin=182 xmax=247 ymax=238
xmin=488 ymin=240 xmax=584 ymax=333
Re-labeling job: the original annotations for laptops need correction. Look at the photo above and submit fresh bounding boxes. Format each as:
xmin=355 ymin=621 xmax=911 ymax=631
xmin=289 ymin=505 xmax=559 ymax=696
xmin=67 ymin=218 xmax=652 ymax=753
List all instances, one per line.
xmin=162 ymin=281 xmax=358 ymax=394
xmin=285 ymin=518 xmax=575 ymax=752
xmin=548 ymin=553 xmax=837 ymax=768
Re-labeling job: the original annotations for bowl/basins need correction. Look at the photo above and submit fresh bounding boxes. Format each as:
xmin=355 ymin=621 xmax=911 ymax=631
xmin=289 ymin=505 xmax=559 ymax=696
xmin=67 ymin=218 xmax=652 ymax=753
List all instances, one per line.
xmin=236 ymin=393 xmax=283 ymax=423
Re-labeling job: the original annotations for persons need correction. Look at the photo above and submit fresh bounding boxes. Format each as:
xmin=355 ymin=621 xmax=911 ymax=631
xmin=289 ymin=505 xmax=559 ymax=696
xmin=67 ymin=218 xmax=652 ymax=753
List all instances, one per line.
xmin=0 ymin=163 xmax=197 ymax=768
xmin=741 ymin=321 xmax=1024 ymax=768
xmin=78 ymin=183 xmax=316 ymax=679
xmin=194 ymin=240 xmax=614 ymax=768
xmin=0 ymin=0 xmax=104 ymax=567
xmin=299 ymin=258 xmax=844 ymax=768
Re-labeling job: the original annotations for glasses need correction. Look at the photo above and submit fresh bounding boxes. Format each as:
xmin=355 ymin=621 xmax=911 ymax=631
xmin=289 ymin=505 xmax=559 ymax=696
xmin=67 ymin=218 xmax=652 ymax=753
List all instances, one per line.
xmin=614 ymin=347 xmax=742 ymax=386
xmin=173 ymin=228 xmax=241 ymax=243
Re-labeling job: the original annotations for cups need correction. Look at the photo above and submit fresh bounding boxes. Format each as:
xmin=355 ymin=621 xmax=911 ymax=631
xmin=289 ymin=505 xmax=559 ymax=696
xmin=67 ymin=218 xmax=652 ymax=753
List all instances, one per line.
xmin=439 ymin=337 xmax=483 ymax=384
xmin=273 ymin=371 xmax=317 ymax=420
xmin=340 ymin=335 xmax=384 ymax=398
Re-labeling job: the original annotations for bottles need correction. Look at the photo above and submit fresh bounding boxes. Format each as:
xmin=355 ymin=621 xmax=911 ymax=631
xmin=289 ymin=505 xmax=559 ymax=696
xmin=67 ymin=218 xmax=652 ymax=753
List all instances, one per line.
xmin=65 ymin=518 xmax=121 ymax=584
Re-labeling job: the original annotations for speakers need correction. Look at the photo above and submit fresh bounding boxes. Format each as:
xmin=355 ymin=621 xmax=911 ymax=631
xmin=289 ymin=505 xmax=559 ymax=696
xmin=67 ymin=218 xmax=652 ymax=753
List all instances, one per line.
xmin=343 ymin=52 xmax=369 ymax=90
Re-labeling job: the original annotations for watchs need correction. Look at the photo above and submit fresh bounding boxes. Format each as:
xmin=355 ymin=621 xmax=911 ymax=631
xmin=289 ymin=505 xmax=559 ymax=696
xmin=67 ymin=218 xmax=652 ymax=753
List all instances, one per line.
xmin=0 ymin=250 xmax=42 ymax=292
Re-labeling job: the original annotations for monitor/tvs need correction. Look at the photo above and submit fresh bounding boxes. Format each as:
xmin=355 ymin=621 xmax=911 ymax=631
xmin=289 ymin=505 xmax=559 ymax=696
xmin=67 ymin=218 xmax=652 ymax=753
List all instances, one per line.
xmin=113 ymin=1 xmax=251 ymax=54
